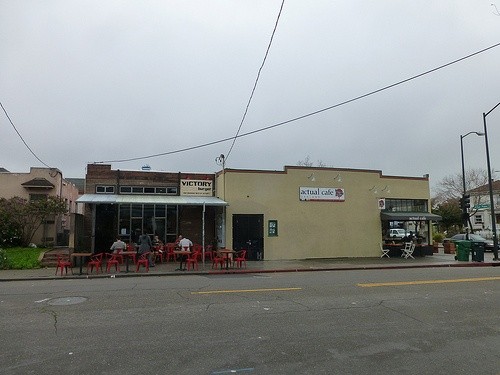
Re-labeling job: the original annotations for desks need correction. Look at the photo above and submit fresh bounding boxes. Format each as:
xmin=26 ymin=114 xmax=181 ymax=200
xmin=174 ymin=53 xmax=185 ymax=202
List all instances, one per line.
xmin=174 ymin=250 xmax=191 ymax=271
xmin=385 ymin=242 xmax=402 ymax=259
xmin=70 ymin=252 xmax=93 ymax=275
xmin=218 ymin=250 xmax=236 ymax=270
xmin=118 ymin=251 xmax=137 ymax=273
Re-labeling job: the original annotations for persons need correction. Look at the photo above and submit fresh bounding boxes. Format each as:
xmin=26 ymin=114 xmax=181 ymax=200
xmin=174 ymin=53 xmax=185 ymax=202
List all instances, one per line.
xmin=175 ymin=234 xmax=193 ymax=261
xmin=408 ymin=231 xmax=414 ymax=238
xmin=256 ymin=234 xmax=264 ymax=260
xmin=110 ymin=236 xmax=126 ymax=262
xmin=137 ymin=230 xmax=153 ymax=267
xmin=152 ymin=235 xmax=162 ymax=246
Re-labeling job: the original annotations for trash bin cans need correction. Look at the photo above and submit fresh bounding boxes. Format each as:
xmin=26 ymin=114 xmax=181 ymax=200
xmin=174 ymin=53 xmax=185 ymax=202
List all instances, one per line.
xmin=471 ymin=241 xmax=487 ymax=262
xmin=455 ymin=240 xmax=471 ymax=261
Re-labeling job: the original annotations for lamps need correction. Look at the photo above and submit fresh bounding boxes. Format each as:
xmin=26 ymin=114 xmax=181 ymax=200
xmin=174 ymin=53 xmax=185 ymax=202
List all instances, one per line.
xmin=369 ymin=186 xmax=379 ymax=195
xmin=381 ymin=184 xmax=391 ymax=195
xmin=334 ymin=174 xmax=342 ymax=183
xmin=308 ymin=172 xmax=315 ymax=181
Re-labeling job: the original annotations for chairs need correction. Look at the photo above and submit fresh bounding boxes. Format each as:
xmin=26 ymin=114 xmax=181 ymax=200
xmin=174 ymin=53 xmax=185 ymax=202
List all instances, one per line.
xmin=105 ymin=243 xmax=233 ymax=272
xmin=233 ymin=250 xmax=246 ymax=269
xmin=86 ymin=253 xmax=104 ymax=275
xmin=54 ymin=253 xmax=73 ymax=276
xmin=379 ymin=243 xmax=391 ymax=258
xmin=401 ymin=241 xmax=415 ymax=260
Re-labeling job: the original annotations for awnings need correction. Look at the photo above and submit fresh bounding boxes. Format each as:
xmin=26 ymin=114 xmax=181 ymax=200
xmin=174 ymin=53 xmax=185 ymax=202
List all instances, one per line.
xmin=382 ymin=212 xmax=442 ymax=220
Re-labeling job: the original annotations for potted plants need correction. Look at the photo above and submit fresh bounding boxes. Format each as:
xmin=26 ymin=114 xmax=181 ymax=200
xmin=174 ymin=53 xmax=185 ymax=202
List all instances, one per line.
xmin=444 ymin=240 xmax=456 ymax=254
